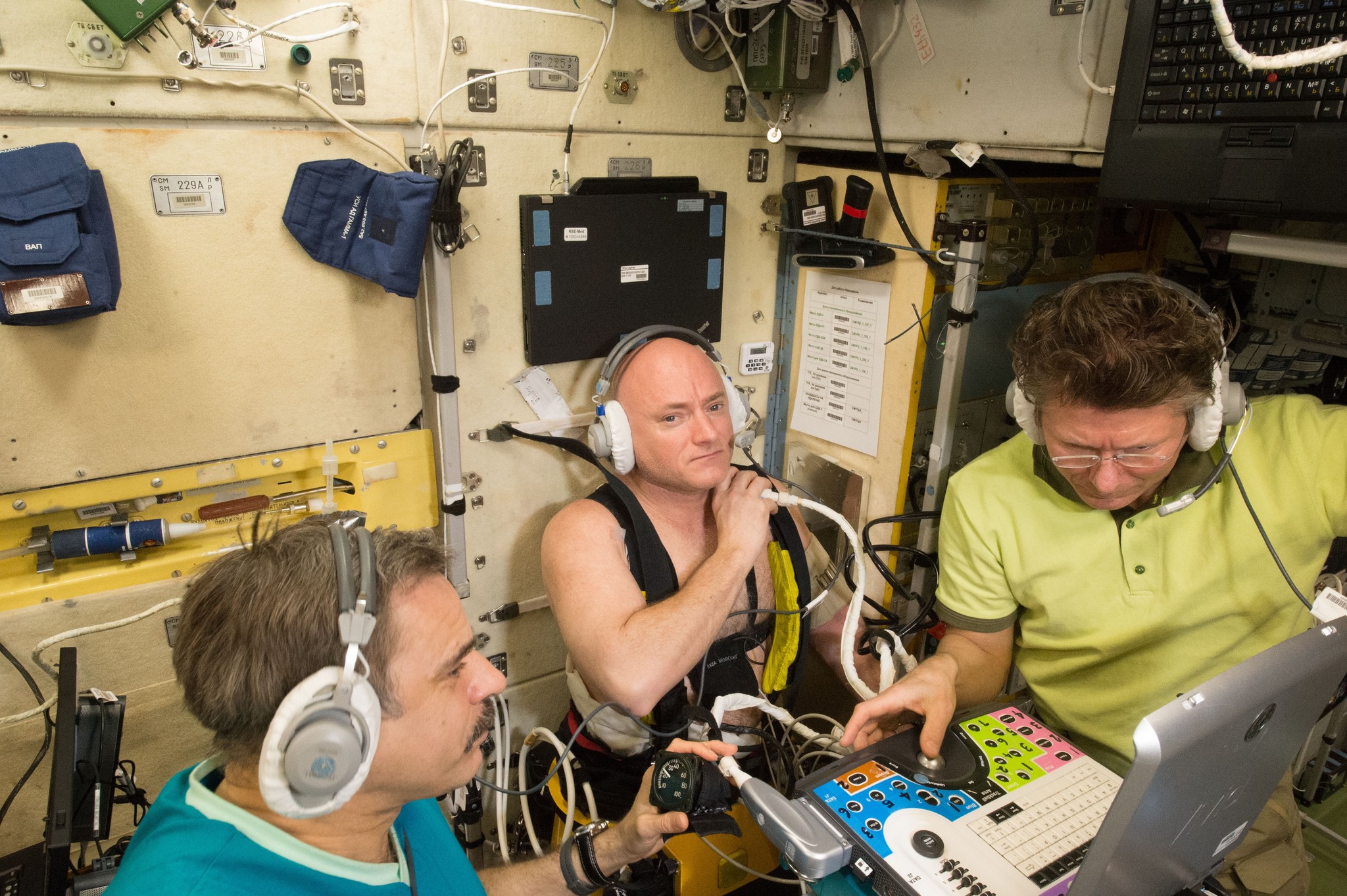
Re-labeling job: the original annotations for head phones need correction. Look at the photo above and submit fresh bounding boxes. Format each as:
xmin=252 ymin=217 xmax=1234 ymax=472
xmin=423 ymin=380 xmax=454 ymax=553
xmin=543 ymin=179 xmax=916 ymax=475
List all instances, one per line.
xmin=1004 ymin=271 xmax=1245 ymax=454
xmin=587 ymin=325 xmax=751 ymax=477
xmin=259 ymin=523 xmax=382 ymax=816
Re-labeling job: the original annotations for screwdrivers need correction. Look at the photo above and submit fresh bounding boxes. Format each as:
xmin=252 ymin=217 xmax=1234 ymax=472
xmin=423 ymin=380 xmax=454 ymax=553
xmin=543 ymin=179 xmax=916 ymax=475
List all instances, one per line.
xmin=198 ymin=484 xmax=354 ymax=520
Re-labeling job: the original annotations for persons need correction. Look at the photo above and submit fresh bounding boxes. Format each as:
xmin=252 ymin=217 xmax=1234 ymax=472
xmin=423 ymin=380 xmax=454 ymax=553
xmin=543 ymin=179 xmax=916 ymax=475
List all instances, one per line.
xmin=539 ymin=324 xmax=893 ymax=896
xmin=840 ymin=266 xmax=1347 ymax=896
xmin=97 ymin=509 xmax=737 ymax=896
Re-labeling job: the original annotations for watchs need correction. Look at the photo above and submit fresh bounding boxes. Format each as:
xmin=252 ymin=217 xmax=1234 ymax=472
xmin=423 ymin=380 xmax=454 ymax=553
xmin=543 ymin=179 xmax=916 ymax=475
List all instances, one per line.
xmin=573 ymin=818 xmax=621 ymax=887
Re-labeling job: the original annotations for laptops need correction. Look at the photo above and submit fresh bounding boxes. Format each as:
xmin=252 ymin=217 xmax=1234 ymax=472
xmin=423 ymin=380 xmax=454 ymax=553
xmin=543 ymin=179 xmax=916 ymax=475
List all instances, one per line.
xmin=793 ymin=612 xmax=1347 ymax=895
xmin=1097 ymin=0 xmax=1347 ymax=217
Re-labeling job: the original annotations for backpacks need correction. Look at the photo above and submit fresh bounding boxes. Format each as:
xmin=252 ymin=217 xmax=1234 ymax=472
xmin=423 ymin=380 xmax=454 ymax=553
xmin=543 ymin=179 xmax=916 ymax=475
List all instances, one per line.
xmin=0 ymin=145 xmax=120 ymax=326
xmin=283 ymin=158 xmax=438 ymax=298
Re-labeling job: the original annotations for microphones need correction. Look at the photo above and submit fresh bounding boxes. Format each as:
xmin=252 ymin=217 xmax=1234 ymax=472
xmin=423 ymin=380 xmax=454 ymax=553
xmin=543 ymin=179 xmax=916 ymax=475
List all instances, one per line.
xmin=735 ymin=406 xmax=763 ymax=449
xmin=1155 ymin=396 xmax=1250 ymax=517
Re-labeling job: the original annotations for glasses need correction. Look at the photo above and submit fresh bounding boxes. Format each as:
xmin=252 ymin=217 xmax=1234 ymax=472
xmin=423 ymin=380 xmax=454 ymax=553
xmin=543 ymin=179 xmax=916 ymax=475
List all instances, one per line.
xmin=1052 ymin=436 xmax=1187 ymax=468
